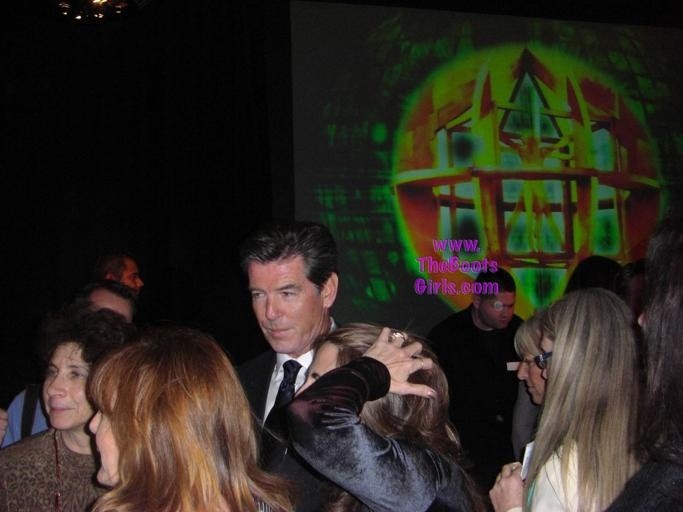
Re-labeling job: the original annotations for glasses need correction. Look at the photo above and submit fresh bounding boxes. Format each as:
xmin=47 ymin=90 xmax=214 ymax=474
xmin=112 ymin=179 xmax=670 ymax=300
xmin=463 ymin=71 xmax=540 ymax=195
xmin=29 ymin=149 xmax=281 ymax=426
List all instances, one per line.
xmin=534 ymin=352 xmax=552 ymax=368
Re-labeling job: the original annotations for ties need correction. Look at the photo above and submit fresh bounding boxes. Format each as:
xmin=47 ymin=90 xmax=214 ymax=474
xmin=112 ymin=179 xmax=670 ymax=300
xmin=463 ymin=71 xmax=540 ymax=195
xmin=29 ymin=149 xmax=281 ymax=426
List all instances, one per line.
xmin=260 ymin=360 xmax=301 ymax=466
